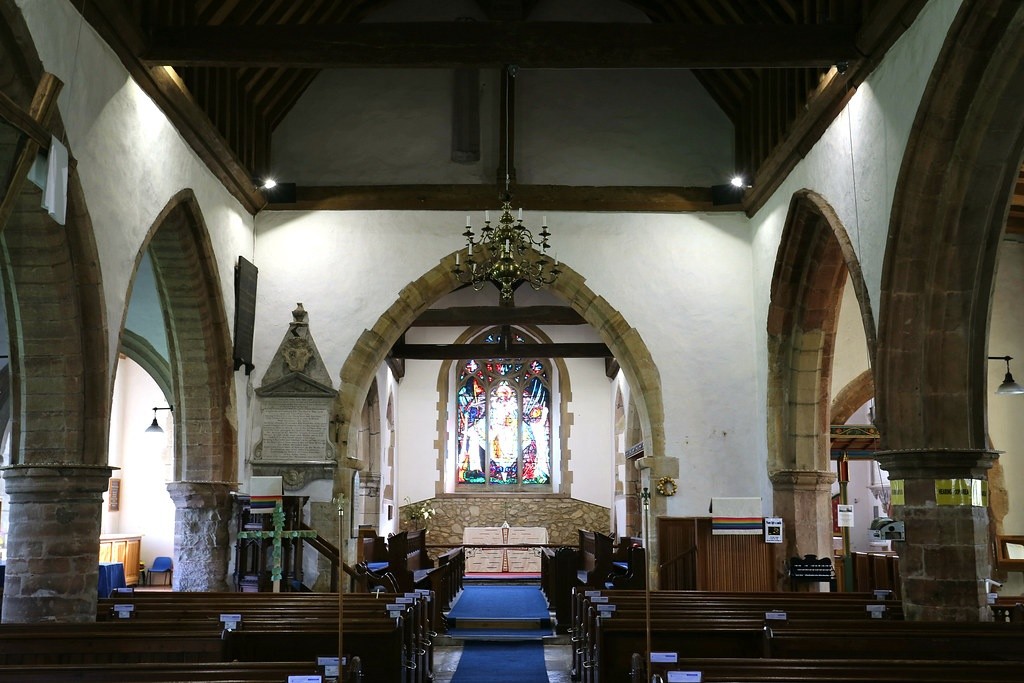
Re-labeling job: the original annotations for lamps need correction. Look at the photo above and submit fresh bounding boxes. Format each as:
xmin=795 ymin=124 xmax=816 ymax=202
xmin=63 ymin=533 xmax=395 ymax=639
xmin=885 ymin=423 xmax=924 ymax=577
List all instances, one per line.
xmin=253 ymin=178 xmax=277 ymax=192
xmin=730 ymin=175 xmax=756 ymax=190
xmin=452 ymin=60 xmax=561 ymax=304
xmin=987 ymin=356 xmax=1024 ymax=396
xmin=143 ymin=405 xmax=173 ymax=432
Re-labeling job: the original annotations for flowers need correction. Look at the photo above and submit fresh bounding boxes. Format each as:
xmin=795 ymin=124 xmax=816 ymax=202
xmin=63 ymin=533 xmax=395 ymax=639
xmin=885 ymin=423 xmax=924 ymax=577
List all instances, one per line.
xmin=656 ymin=476 xmax=679 ymax=497
xmin=403 ymin=496 xmax=436 ymax=533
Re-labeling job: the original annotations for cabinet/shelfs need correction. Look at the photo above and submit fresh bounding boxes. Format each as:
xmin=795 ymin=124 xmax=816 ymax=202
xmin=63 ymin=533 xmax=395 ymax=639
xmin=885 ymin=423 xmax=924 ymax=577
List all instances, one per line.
xmin=101 ymin=534 xmax=142 ymax=592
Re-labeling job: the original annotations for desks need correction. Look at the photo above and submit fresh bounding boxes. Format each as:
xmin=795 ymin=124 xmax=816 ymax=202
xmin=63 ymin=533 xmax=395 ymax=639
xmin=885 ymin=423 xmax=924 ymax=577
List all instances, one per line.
xmin=99 ymin=564 xmax=127 ymax=596
xmin=463 ymin=526 xmax=549 ymax=574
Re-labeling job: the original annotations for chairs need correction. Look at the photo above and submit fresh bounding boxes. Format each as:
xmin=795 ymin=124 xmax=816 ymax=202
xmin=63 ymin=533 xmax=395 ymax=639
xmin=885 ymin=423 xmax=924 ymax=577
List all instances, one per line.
xmin=144 ymin=557 xmax=173 ymax=586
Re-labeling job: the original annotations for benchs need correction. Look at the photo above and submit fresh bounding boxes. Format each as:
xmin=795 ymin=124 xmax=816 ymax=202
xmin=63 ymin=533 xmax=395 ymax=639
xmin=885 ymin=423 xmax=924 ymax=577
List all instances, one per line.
xmin=539 ymin=529 xmax=1024 ymax=682
xmin=0 ymin=526 xmax=465 ymax=683
